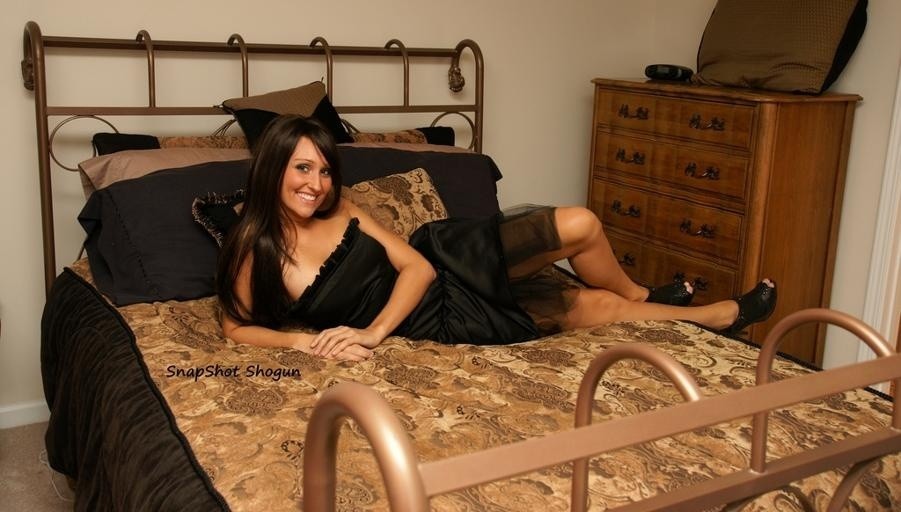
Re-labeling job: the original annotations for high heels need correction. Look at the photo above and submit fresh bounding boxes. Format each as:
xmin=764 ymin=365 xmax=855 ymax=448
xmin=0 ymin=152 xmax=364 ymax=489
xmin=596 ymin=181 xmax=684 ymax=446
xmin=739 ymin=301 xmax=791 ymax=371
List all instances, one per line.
xmin=641 ymin=279 xmax=696 ymax=308
xmin=715 ymin=278 xmax=778 ymax=339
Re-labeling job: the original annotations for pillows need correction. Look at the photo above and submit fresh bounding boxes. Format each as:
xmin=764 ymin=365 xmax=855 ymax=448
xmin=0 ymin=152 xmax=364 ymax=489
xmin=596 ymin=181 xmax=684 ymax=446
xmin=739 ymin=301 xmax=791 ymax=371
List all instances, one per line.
xmin=687 ymin=1 xmax=872 ymax=98
xmin=61 ymin=74 xmax=512 ymax=308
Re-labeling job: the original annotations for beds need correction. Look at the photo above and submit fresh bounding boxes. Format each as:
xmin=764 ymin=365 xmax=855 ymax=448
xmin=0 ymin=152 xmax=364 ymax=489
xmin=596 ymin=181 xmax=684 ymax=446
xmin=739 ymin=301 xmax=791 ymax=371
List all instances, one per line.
xmin=17 ymin=21 xmax=901 ymax=511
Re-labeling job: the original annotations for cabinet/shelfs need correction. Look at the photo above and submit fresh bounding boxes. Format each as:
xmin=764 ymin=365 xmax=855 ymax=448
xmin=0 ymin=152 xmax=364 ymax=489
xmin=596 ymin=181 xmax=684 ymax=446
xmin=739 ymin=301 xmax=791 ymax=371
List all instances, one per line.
xmin=585 ymin=66 xmax=866 ymax=372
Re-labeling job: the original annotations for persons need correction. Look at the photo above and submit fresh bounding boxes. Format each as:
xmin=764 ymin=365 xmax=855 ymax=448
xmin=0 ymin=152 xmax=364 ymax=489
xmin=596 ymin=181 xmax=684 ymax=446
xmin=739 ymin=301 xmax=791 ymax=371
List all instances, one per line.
xmin=213 ymin=113 xmax=779 ymax=364
